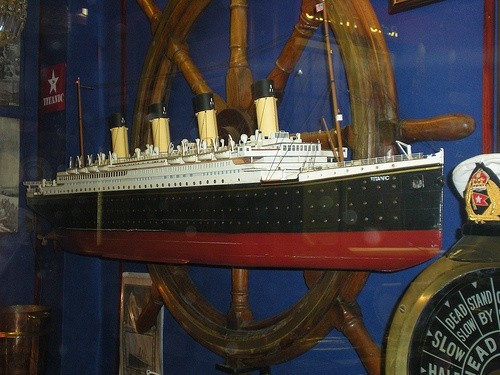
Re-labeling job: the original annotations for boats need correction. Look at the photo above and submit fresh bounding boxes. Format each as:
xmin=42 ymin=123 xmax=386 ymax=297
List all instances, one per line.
xmin=23 ymin=79 xmax=445 ymax=272
